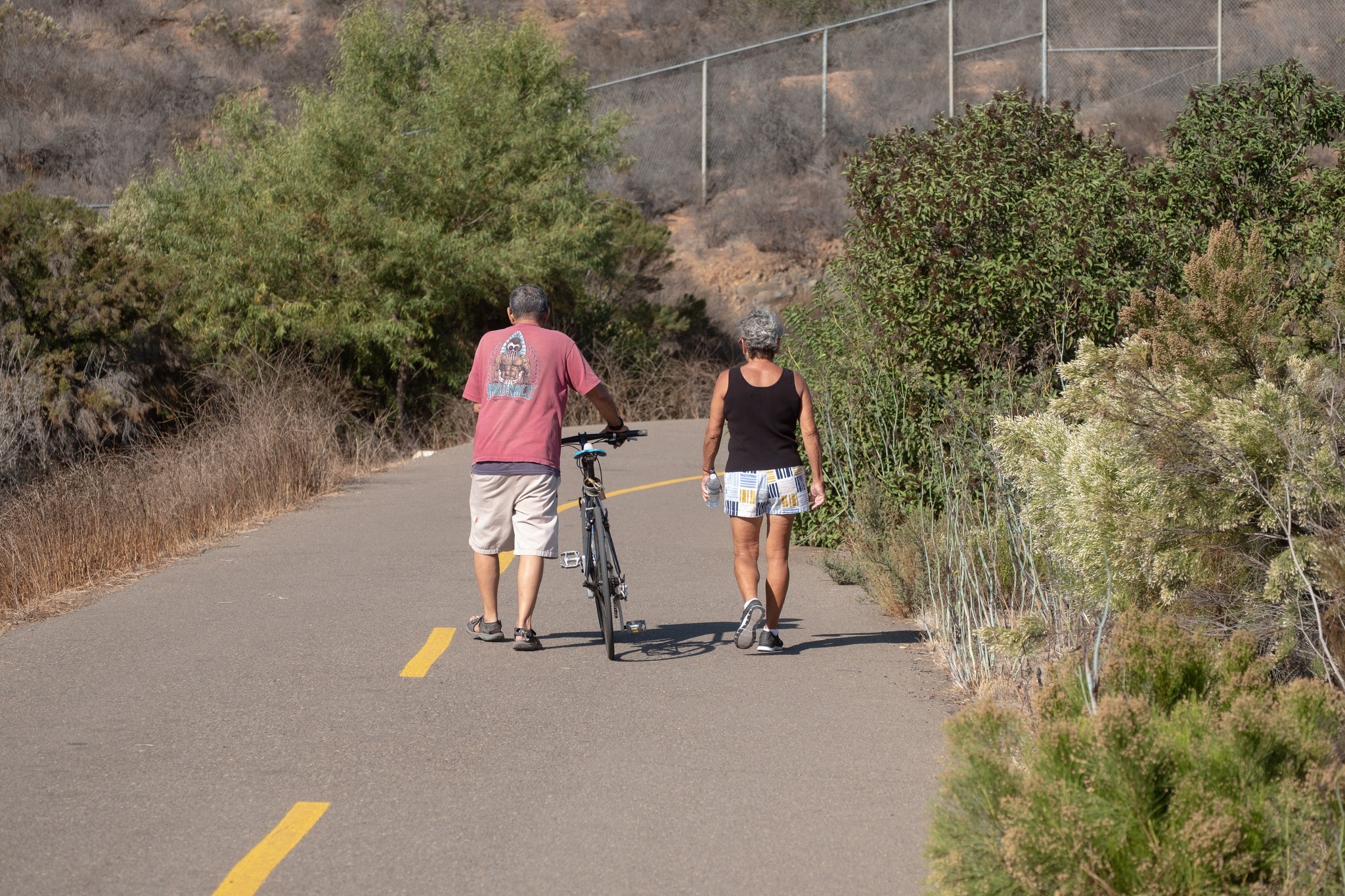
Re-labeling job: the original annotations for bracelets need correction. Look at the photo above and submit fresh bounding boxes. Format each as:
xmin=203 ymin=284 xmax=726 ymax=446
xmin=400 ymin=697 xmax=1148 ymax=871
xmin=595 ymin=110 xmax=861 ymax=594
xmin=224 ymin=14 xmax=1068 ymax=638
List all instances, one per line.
xmin=608 ymin=417 xmax=624 ymax=431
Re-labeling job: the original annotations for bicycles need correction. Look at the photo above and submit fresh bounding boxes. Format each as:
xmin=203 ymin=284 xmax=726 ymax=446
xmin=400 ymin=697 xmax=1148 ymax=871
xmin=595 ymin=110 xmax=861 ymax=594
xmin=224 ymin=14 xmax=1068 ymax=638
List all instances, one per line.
xmin=560 ymin=429 xmax=649 ymax=660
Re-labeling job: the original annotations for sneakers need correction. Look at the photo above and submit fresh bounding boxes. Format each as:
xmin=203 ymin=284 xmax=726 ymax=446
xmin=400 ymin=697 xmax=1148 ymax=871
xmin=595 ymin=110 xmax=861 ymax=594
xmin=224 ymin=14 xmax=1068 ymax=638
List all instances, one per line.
xmin=734 ymin=601 xmax=766 ymax=649
xmin=757 ymin=628 xmax=783 ymax=653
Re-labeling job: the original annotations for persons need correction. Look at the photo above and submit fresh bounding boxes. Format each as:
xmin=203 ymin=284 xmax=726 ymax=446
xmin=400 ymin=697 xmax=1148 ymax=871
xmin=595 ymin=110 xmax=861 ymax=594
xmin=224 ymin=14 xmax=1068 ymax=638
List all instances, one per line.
xmin=462 ymin=284 xmax=630 ymax=651
xmin=701 ymin=308 xmax=824 ymax=652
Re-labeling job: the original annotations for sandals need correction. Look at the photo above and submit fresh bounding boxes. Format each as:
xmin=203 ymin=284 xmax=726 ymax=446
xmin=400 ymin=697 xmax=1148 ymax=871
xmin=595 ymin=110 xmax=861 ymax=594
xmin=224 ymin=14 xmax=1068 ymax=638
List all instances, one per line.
xmin=465 ymin=612 xmax=505 ymax=642
xmin=513 ymin=629 xmax=543 ymax=650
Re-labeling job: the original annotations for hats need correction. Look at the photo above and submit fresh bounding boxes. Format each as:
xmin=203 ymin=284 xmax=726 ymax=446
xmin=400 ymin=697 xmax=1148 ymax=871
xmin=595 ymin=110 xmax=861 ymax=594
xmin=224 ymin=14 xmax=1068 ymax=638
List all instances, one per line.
xmin=738 ymin=337 xmax=743 ymax=344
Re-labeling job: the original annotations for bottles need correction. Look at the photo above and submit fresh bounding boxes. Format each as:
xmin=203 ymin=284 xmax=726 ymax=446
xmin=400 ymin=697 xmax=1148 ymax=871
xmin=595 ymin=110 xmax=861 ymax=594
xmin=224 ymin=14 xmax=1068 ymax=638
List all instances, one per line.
xmin=591 ymin=526 xmax=606 ymax=556
xmin=706 ymin=474 xmax=720 ymax=509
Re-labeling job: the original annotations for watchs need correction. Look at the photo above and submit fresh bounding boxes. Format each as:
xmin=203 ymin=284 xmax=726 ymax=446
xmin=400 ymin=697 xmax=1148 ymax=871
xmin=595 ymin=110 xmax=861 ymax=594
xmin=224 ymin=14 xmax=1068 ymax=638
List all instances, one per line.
xmin=702 ymin=465 xmax=715 ymax=473
